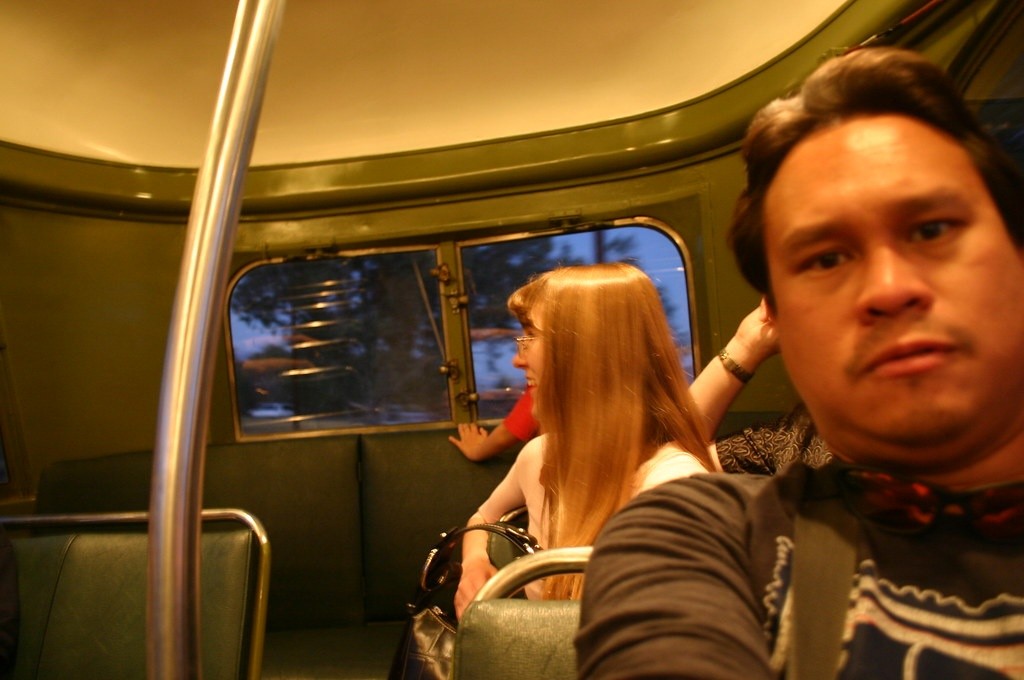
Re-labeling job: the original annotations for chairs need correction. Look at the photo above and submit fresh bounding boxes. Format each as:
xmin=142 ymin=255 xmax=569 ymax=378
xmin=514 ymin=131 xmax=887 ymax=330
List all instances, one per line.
xmin=452 ymin=506 xmax=596 ymax=679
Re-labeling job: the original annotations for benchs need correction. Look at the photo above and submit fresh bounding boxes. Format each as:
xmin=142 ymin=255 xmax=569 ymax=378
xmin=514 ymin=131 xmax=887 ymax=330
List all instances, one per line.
xmin=1 ymin=508 xmax=270 ymax=680
xmin=28 ymin=411 xmax=793 ymax=680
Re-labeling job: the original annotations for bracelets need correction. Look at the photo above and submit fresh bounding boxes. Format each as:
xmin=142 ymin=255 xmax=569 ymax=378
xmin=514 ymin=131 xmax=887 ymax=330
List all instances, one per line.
xmin=717 ymin=347 xmax=755 ymax=385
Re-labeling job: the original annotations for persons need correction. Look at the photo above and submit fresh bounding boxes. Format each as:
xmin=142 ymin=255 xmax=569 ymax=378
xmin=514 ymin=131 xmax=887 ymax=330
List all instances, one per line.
xmin=449 ymin=47 xmax=1024 ymax=680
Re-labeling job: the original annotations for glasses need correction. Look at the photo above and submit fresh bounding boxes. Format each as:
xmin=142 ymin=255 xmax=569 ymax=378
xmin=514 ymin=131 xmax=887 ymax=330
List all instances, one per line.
xmin=515 ymin=335 xmax=541 ymax=358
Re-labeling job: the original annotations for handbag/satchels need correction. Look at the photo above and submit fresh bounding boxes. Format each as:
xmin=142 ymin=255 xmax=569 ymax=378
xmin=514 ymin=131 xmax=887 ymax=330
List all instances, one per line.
xmin=389 ymin=523 xmax=539 ymax=680
